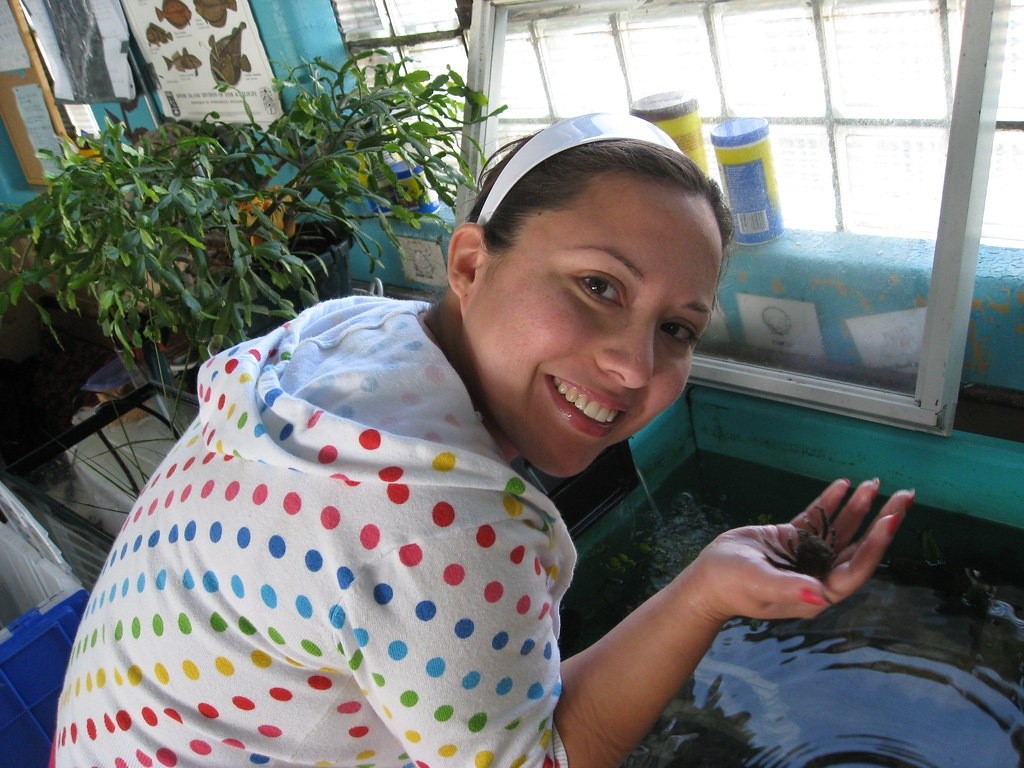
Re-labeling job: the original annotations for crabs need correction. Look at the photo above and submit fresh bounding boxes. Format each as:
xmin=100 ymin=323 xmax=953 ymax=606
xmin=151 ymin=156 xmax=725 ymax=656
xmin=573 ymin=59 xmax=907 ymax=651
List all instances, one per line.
xmin=764 ymin=506 xmax=839 ymax=583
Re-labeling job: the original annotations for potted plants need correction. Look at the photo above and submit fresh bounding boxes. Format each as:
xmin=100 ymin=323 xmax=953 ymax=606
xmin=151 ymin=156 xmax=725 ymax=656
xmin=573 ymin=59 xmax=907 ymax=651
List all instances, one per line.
xmin=0 ymin=51 xmax=507 ymax=363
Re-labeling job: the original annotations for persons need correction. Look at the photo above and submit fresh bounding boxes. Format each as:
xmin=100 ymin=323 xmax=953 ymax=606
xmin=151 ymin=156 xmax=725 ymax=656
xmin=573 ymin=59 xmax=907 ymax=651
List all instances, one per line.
xmin=48 ymin=113 xmax=915 ymax=768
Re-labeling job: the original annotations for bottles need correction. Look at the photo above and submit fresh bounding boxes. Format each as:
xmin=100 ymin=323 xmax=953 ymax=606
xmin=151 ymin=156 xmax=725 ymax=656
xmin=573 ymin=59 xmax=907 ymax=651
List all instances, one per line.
xmin=710 ymin=116 xmax=786 ymax=245
xmin=391 ymin=159 xmax=441 ymax=215
xmin=346 ymin=137 xmax=399 ymax=216
xmin=630 ymin=91 xmax=711 ymax=178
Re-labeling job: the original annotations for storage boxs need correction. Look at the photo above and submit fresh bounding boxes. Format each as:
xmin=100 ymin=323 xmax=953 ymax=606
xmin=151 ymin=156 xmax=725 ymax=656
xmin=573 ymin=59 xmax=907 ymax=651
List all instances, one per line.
xmin=1 ymin=523 xmax=92 ymax=768
